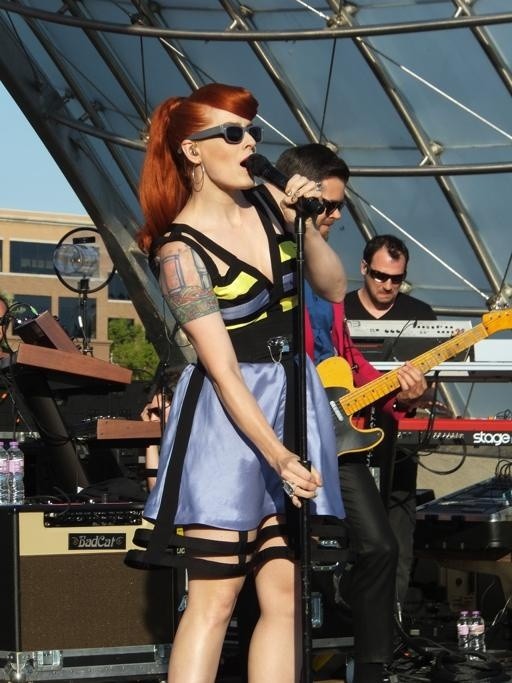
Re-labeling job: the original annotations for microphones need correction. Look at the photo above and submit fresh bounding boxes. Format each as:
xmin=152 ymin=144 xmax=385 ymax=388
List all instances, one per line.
xmin=245 ymin=153 xmax=325 ymax=215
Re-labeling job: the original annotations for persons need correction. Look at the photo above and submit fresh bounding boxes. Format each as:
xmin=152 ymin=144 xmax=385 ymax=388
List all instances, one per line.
xmin=1 ymin=298 xmax=16 ymax=359
xmin=137 ymin=84 xmax=350 ymax=682
xmin=276 ymin=142 xmax=428 ymax=682
xmin=345 ymin=234 xmax=443 ymax=613
xmin=142 ymin=386 xmax=173 ymax=490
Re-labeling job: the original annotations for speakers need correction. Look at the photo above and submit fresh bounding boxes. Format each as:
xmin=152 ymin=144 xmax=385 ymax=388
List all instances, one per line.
xmin=0 ymin=505 xmax=179 ymax=683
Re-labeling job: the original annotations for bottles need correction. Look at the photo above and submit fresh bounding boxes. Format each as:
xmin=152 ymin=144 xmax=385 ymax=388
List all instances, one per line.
xmin=455 ymin=609 xmax=472 ymax=660
xmin=467 ymin=610 xmax=487 ymax=661
xmin=1 ymin=441 xmax=12 ymax=503
xmin=8 ymin=441 xmax=25 ymax=504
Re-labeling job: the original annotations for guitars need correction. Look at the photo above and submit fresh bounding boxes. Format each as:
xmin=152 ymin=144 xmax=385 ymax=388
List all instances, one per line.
xmin=314 ymin=310 xmax=512 ymax=460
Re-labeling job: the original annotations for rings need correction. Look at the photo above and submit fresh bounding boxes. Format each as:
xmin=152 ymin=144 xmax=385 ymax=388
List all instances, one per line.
xmin=282 ymin=480 xmax=298 ymax=497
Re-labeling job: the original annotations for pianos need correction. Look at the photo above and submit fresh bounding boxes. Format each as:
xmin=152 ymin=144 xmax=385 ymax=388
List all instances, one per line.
xmin=358 ymin=413 xmax=512 ymax=461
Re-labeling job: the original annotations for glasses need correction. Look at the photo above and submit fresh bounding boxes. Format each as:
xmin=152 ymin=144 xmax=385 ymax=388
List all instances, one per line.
xmin=367 ymin=265 xmax=407 ymax=283
xmin=323 ymin=197 xmax=344 ymax=214
xmin=186 ymin=124 xmax=265 ymax=144
